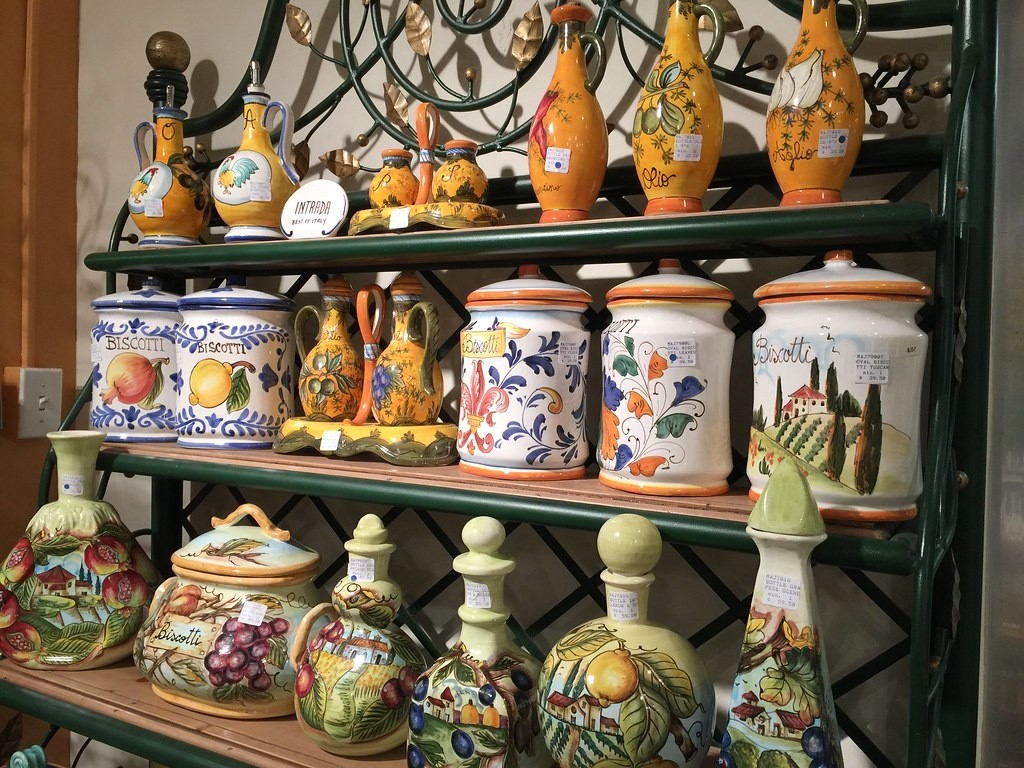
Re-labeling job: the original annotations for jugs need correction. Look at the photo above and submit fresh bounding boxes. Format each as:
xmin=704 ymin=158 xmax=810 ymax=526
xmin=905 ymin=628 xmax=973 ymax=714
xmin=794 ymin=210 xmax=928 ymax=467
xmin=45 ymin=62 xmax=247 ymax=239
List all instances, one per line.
xmin=211 ymin=60 xmax=303 ymax=243
xmin=766 ymin=0 xmax=869 ymax=208
xmin=368 ymin=268 xmax=443 ymax=426
xmin=527 ymin=0 xmax=608 ymax=222
xmin=293 ymin=272 xmax=362 ymax=423
xmin=633 ymin=0 xmax=725 ymax=216
xmin=127 ymin=83 xmax=214 ymax=248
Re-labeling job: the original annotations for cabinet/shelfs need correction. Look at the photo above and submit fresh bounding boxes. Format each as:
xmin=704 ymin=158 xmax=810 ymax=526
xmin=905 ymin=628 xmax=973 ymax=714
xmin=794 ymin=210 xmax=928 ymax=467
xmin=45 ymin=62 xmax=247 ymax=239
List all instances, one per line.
xmin=0 ymin=0 xmax=997 ymax=768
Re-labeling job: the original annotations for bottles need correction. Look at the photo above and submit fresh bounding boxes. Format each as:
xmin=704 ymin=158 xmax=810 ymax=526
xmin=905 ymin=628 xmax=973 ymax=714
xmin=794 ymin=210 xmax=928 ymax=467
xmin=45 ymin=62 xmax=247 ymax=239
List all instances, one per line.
xmin=409 ymin=514 xmax=554 ymax=768
xmin=0 ymin=432 xmax=167 ymax=670
xmin=720 ymin=458 xmax=845 ymax=768
xmin=538 ymin=513 xmax=716 ymax=767
xmin=290 ymin=514 xmax=429 ymax=757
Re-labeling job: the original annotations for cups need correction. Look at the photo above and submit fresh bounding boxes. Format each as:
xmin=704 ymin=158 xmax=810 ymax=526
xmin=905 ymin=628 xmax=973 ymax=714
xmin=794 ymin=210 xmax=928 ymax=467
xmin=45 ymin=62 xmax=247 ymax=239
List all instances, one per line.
xmin=596 ymin=259 xmax=735 ymax=496
xmin=746 ymin=249 xmax=934 ymax=523
xmin=172 ymin=270 xmax=298 ymax=450
xmin=454 ymin=264 xmax=593 ymax=483
xmin=89 ymin=280 xmax=186 ymax=443
